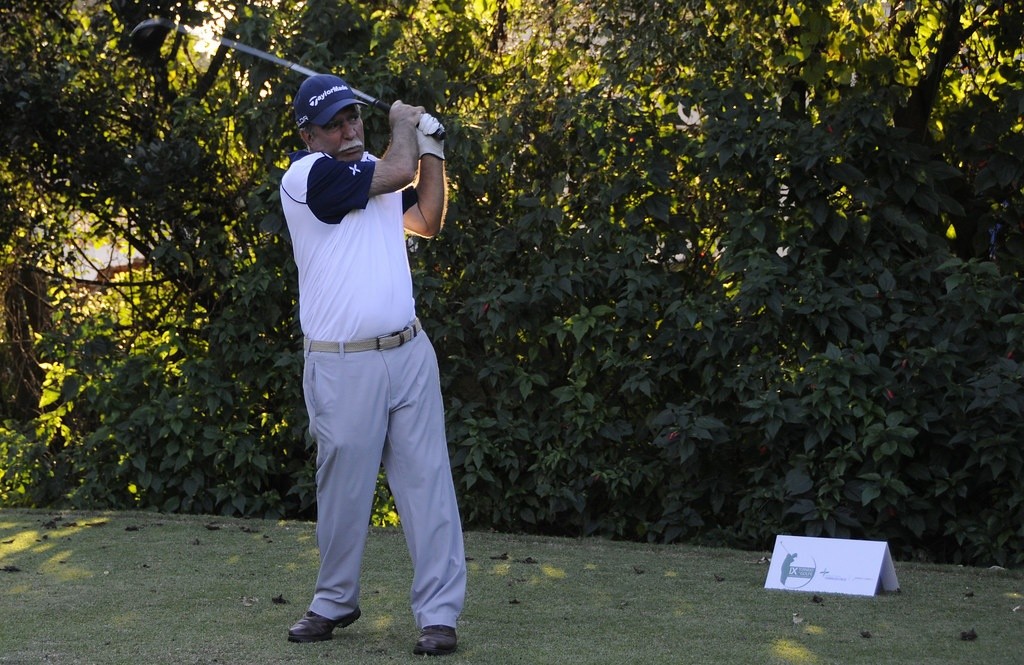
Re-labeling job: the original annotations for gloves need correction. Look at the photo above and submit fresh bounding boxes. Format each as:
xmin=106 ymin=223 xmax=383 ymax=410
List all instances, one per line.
xmin=415 ymin=113 xmax=446 ymax=161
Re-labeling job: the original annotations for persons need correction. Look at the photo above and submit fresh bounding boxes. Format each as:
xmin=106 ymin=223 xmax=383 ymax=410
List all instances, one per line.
xmin=279 ymin=73 xmax=466 ymax=656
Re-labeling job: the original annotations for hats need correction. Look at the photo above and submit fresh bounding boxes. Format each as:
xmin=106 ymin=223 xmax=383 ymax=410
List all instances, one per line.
xmin=293 ymin=75 xmax=367 ymax=129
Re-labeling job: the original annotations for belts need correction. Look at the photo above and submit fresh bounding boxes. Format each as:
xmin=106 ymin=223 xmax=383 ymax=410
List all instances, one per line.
xmin=303 ymin=317 xmax=422 ymax=353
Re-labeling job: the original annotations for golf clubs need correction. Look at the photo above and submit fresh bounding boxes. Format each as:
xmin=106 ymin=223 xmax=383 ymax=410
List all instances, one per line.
xmin=123 ymin=16 xmax=445 ymax=139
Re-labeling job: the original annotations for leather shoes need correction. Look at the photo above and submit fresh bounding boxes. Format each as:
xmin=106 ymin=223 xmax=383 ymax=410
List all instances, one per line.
xmin=288 ymin=605 xmax=361 ymax=643
xmin=413 ymin=624 xmax=459 ymax=655
